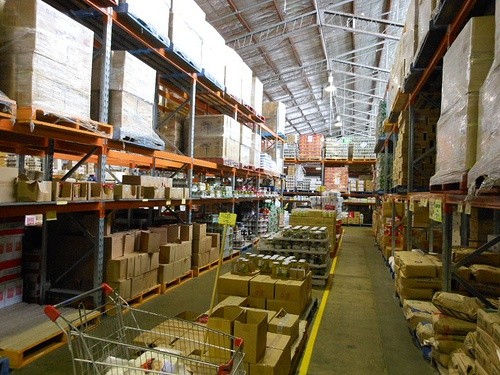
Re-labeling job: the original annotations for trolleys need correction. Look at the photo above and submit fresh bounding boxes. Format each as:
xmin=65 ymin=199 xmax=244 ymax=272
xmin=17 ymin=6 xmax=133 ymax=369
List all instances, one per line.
xmin=45 ymin=283 xmax=247 ymax=375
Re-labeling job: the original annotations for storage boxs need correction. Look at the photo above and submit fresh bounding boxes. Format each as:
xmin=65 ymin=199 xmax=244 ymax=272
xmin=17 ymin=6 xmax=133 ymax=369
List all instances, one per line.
xmin=1 ymin=1 xmax=499 ymax=373
